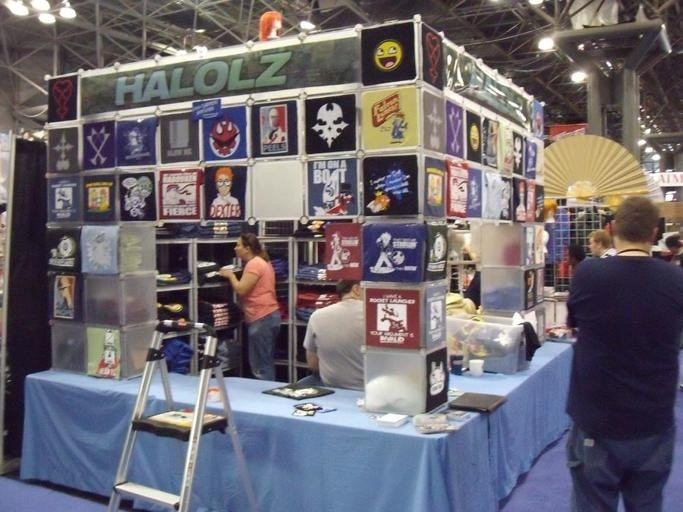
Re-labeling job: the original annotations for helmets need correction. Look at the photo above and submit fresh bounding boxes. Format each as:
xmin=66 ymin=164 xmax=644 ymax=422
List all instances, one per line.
xmin=57 ymin=278 xmax=71 ymax=289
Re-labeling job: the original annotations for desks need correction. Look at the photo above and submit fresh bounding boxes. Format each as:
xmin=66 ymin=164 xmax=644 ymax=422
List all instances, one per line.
xmin=447 ymin=340 xmax=574 ymax=499
xmin=17 ymin=368 xmax=495 ymax=512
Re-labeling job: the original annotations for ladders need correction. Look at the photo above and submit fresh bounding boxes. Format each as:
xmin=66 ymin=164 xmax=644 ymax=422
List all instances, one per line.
xmin=106 ymin=320 xmax=259 ymax=512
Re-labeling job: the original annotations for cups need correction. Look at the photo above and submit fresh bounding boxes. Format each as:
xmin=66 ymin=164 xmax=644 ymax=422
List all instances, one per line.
xmin=468 ymin=359 xmax=484 ymax=375
xmin=449 ymin=353 xmax=462 ymax=374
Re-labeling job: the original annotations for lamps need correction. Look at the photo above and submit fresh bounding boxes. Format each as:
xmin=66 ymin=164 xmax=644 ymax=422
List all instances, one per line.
xmin=3 ymin=1 xmax=78 ymax=30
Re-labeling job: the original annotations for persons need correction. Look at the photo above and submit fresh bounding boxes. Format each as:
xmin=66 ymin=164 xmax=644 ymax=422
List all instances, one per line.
xmin=515 ymin=181 xmax=527 ymax=220
xmin=57 ymin=277 xmax=74 ymax=311
xmin=219 ymin=233 xmax=282 ymax=379
xmin=303 ymin=282 xmax=366 ymax=389
xmin=212 ymin=166 xmax=239 ymax=217
xmin=567 ymin=195 xmax=683 ymax=512
xmin=263 ymin=108 xmax=284 ymax=142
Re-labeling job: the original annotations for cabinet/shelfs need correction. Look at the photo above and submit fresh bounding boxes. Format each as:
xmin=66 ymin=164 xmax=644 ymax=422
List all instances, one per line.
xmin=76 ymin=220 xmax=159 ymax=384
xmin=478 ymin=220 xmax=538 ymax=320
xmin=44 ymin=227 xmax=88 ymax=376
xmin=158 ymin=235 xmax=343 ymax=392
xmin=356 ymin=220 xmax=451 ymax=419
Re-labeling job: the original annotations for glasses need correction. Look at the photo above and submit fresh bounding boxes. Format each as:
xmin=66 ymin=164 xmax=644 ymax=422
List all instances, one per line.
xmin=216 ymin=180 xmax=231 ymax=187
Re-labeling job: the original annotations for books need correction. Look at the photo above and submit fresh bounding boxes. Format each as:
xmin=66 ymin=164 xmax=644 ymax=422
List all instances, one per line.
xmin=449 ymin=393 xmax=508 ymax=414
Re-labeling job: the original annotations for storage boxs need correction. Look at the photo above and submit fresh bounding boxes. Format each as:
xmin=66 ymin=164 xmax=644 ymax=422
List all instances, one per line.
xmin=445 ymin=311 xmax=536 ymax=376
